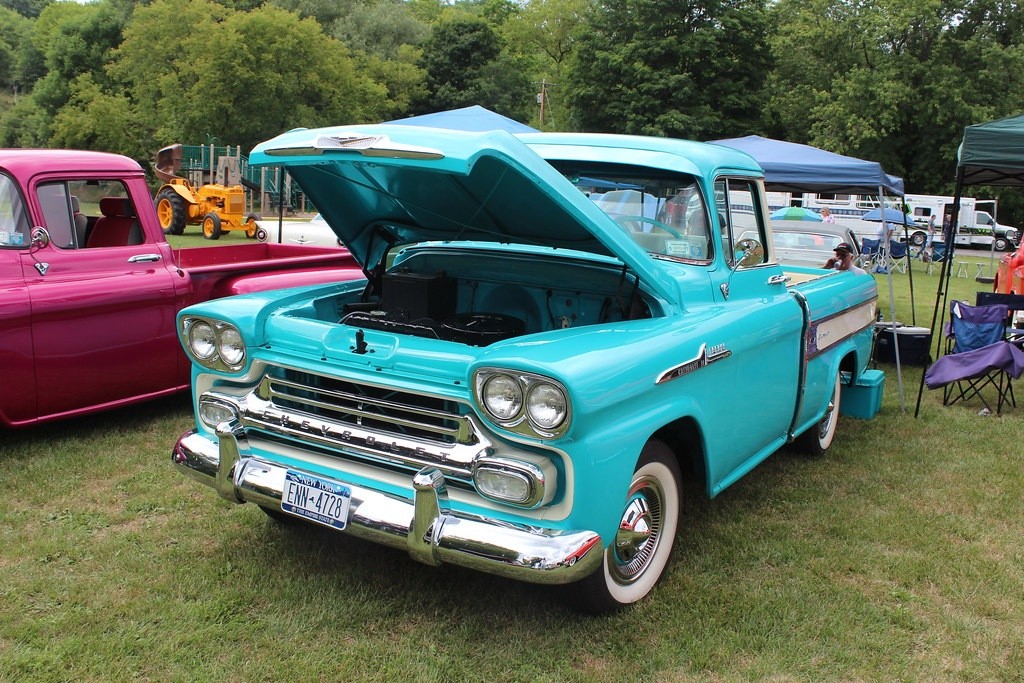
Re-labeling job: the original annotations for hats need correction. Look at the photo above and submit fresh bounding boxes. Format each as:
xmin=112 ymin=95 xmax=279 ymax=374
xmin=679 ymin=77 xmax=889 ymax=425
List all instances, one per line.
xmin=832 ymin=243 xmax=853 ymax=255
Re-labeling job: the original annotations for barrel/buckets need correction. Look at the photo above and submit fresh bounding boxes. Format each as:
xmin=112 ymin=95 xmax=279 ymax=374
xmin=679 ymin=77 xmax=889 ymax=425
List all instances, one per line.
xmin=873 ymin=320 xmax=931 ymax=365
xmin=840 ymin=367 xmax=887 ymax=420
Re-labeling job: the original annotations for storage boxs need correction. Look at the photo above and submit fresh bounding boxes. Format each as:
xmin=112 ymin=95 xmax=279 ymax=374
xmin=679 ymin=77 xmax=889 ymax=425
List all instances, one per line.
xmin=883 ymin=327 xmax=930 ymax=363
xmin=875 ymin=321 xmax=904 ymax=338
xmin=839 ymin=370 xmax=884 ymax=419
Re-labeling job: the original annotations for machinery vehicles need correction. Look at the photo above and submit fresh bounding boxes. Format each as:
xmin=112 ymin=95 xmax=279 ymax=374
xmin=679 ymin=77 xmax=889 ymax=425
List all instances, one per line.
xmin=154 ymin=176 xmax=265 ymax=241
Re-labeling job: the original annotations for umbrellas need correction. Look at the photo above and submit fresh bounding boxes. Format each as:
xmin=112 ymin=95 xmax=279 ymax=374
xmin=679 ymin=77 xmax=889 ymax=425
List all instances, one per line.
xmin=771 ymin=205 xmax=822 ymax=269
xmin=862 ymin=207 xmax=917 ymax=238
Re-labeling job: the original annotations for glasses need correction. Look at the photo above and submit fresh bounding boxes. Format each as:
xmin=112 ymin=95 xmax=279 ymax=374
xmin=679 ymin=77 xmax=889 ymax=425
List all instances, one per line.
xmin=835 ymin=251 xmax=850 ymax=257
xmin=822 ymin=211 xmax=826 ymax=212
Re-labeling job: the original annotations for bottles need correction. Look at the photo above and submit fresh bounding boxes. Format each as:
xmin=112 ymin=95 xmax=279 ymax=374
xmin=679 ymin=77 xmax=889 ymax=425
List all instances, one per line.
xmin=834 ymin=260 xmax=842 ymax=269
xmin=909 ymin=247 xmax=923 ymax=257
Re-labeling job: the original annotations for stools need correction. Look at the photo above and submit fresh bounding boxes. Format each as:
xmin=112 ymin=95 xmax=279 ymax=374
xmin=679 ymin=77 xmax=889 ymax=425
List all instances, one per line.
xmin=974 ymin=262 xmax=986 ymax=279
xmin=958 ymin=261 xmax=969 ymax=278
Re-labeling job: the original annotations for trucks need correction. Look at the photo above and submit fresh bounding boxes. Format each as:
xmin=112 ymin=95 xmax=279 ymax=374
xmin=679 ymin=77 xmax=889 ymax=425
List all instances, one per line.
xmin=685 ymin=182 xmax=906 ymax=247
xmin=896 ymin=193 xmax=1020 ymax=251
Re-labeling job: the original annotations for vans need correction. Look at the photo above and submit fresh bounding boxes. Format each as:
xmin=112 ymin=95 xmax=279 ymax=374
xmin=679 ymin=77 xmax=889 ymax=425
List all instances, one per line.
xmin=596 ymin=188 xmax=667 ymax=233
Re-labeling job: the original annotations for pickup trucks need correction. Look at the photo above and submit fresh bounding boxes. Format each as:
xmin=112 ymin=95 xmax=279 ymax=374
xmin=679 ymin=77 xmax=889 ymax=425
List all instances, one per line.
xmin=169 ymin=127 xmax=889 ymax=604
xmin=0 ymin=147 xmax=366 ymax=428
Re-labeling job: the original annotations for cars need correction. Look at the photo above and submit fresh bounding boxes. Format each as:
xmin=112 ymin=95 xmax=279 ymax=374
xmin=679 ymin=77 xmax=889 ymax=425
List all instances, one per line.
xmin=736 ymin=223 xmax=871 ymax=270
xmin=993 ymin=229 xmax=1024 ymax=344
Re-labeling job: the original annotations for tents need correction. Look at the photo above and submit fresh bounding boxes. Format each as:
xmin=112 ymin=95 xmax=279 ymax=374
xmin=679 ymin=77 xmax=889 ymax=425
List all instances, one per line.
xmin=917 ymin=116 xmax=1024 ymax=418
xmin=693 ymin=135 xmax=916 ymax=414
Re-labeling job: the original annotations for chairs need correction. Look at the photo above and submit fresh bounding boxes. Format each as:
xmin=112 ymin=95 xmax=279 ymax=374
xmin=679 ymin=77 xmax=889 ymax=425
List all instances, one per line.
xmin=915 ymin=299 xmax=1016 ymax=418
xmin=88 ymin=197 xmax=139 ymax=248
xmin=860 ymin=237 xmax=955 ymax=275
xmin=41 ymin=195 xmax=87 ymax=246
xmin=976 ymin=292 xmax=1024 ymax=348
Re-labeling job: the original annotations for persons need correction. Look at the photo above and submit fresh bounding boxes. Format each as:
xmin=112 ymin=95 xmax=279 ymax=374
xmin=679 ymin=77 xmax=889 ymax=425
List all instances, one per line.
xmin=660 ymin=187 xmax=956 ymax=276
